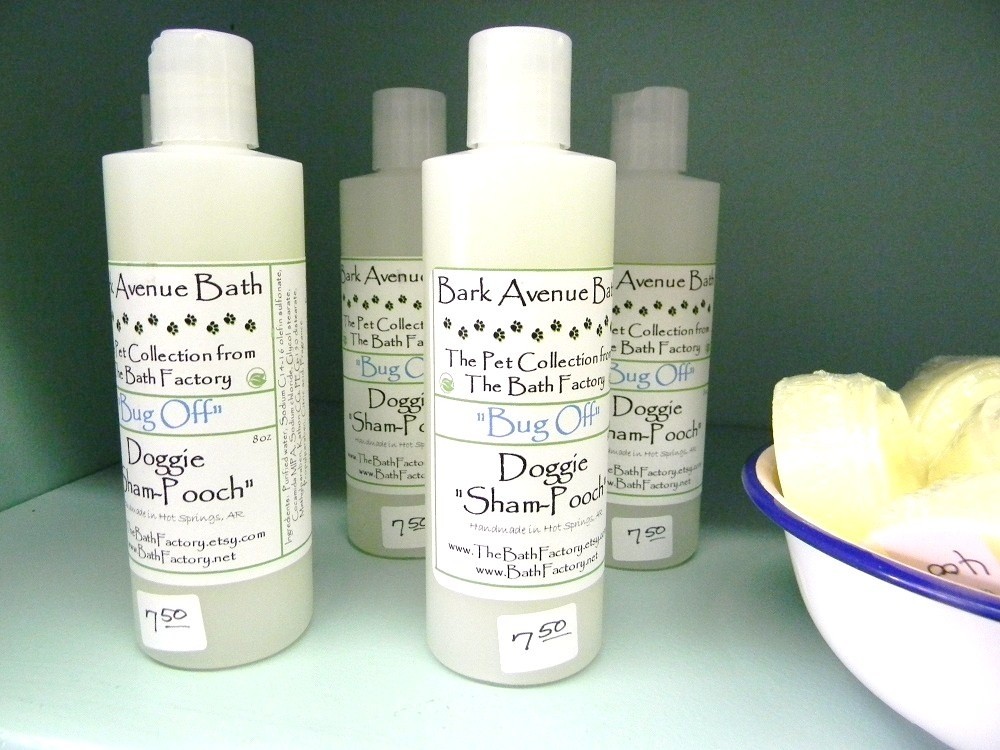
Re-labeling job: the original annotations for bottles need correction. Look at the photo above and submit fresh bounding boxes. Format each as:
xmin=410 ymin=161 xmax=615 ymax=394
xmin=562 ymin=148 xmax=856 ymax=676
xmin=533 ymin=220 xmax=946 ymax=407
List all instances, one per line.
xmin=606 ymin=86 xmax=723 ymax=574
xmin=336 ymin=86 xmax=443 ymax=561
xmin=101 ymin=27 xmax=315 ymax=674
xmin=420 ymin=25 xmax=617 ymax=691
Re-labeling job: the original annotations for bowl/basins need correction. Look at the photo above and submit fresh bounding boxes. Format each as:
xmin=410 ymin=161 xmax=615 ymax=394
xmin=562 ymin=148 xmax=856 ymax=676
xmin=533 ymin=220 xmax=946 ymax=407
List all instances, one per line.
xmin=741 ymin=437 xmax=1000 ymax=750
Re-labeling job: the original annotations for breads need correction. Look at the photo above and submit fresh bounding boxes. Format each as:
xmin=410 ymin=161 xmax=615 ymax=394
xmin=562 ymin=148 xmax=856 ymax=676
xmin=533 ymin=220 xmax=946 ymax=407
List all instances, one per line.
xmin=766 ymin=344 xmax=999 ymax=607
